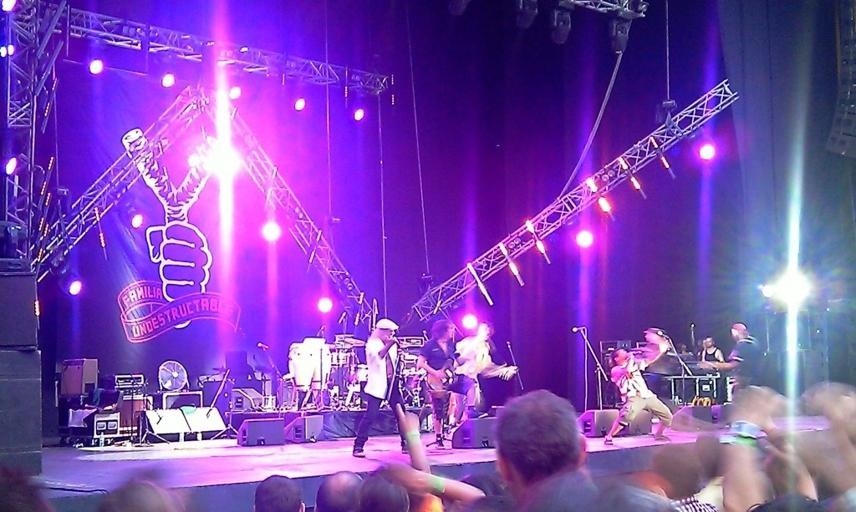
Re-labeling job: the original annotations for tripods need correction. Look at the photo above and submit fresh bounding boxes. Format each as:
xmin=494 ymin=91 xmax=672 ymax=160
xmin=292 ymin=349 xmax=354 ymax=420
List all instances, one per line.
xmin=115 ymin=371 xmax=161 ymax=445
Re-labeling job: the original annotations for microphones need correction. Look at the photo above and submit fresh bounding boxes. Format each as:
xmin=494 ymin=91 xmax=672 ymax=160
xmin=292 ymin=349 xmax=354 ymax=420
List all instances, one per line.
xmin=156 ymin=416 xmax=163 ymax=425
xmin=657 ymin=331 xmax=669 ymax=339
xmin=257 ymin=342 xmax=268 ymax=349
xmin=506 ymin=341 xmax=511 ymax=348
xmin=572 ymin=327 xmax=585 ymax=333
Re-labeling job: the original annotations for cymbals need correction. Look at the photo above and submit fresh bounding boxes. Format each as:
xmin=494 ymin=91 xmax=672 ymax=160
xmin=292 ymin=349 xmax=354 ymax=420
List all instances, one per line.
xmin=336 ymin=333 xmax=354 ymax=337
xmin=344 ymin=338 xmax=366 ymax=347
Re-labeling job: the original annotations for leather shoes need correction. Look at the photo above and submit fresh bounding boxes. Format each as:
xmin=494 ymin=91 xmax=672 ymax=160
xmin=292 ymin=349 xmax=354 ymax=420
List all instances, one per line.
xmin=352 ymin=447 xmax=365 ymax=459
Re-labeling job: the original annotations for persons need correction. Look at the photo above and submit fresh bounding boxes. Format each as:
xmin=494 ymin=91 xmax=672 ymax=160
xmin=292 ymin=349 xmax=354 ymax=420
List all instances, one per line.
xmin=352 ymin=318 xmax=411 ymax=456
xmin=605 ymin=330 xmax=673 ymax=446
xmin=418 ymin=320 xmax=477 ymax=449
xmin=448 ymin=323 xmax=508 ymax=422
xmin=696 ymin=322 xmax=767 ymax=393
xmin=644 ymin=328 xmax=726 ymax=363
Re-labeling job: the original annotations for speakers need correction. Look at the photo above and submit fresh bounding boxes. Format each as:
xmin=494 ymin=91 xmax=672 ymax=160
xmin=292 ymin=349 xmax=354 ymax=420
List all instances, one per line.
xmin=284 ymin=415 xmax=323 ymax=443
xmin=577 ymin=409 xmax=619 ymax=438
xmin=711 ymin=405 xmax=733 ymax=427
xmin=623 ymin=410 xmax=652 ymax=435
xmin=672 ymin=406 xmax=711 ymax=432
xmin=237 ymin=418 xmax=284 ymax=446
xmin=451 ymin=417 xmax=497 ymax=449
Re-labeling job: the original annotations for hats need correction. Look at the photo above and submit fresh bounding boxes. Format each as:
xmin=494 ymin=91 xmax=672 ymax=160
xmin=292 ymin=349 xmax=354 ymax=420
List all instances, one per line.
xmin=374 ymin=317 xmax=399 ymax=331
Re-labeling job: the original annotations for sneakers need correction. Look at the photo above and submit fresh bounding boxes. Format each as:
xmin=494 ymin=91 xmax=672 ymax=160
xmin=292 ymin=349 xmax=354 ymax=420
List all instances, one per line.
xmin=654 ymin=435 xmax=671 ymax=442
xmin=435 ymin=433 xmax=445 ymax=450
xmin=604 ymin=435 xmax=613 ymax=446
xmin=401 ymin=445 xmax=409 ymax=455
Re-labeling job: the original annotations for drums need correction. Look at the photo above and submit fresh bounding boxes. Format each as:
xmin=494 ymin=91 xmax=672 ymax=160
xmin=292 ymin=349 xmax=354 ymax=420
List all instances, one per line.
xmin=288 ymin=343 xmax=331 ymax=390
xmin=347 ymin=364 xmax=367 ymax=382
xmin=330 ymin=353 xmax=349 ymax=368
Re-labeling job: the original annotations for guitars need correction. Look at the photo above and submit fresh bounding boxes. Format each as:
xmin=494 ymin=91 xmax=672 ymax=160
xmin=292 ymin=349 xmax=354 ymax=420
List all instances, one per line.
xmin=427 ymin=355 xmax=468 ymax=391
